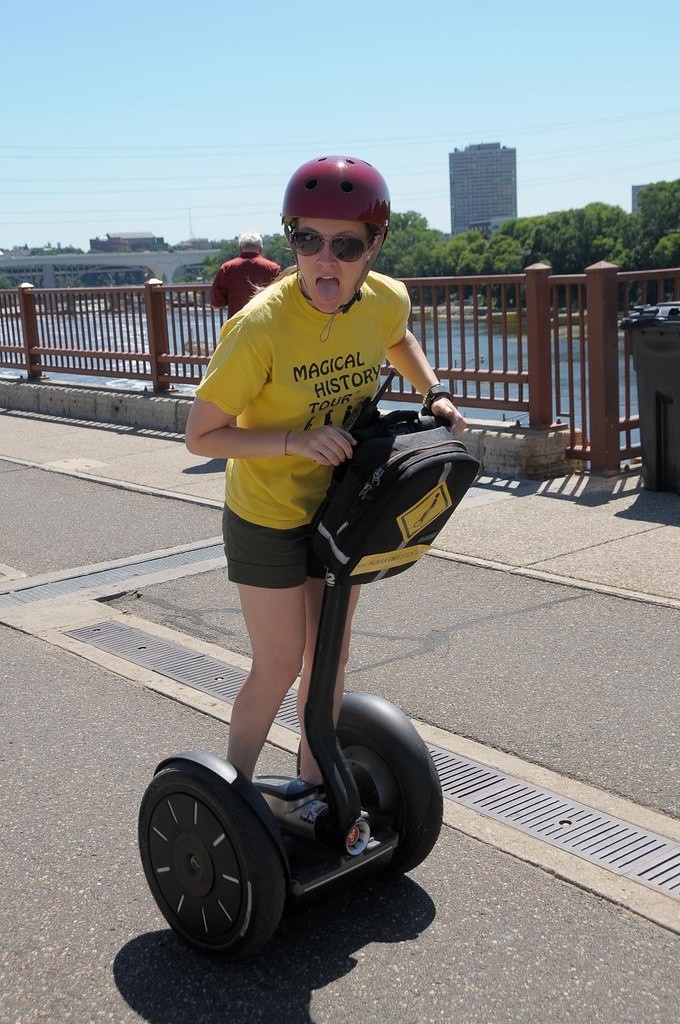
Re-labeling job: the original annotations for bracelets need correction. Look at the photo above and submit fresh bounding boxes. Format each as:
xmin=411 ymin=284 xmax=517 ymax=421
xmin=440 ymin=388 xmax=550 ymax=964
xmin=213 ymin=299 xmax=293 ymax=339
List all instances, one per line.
xmin=422 ymin=383 xmax=453 ymax=412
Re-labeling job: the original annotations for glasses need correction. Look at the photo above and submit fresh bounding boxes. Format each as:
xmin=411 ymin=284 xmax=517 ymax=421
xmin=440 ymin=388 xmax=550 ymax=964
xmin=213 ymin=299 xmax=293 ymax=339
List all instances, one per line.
xmin=288 ymin=229 xmax=380 ymax=263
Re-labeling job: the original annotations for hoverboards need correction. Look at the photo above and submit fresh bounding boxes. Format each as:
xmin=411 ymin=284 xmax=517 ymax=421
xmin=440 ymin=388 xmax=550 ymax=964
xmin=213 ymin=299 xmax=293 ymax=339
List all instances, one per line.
xmin=136 ymin=411 xmax=444 ymax=959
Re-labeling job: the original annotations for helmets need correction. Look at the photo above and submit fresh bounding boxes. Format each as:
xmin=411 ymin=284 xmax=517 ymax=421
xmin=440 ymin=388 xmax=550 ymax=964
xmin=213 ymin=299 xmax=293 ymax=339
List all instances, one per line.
xmin=283 ymin=156 xmax=391 ymax=227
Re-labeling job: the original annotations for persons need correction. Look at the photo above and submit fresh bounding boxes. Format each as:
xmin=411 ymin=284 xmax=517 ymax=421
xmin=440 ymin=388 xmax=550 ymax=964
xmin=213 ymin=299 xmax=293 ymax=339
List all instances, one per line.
xmin=185 ymin=154 xmax=468 ymax=784
xmin=209 ymin=232 xmax=282 ymax=320
